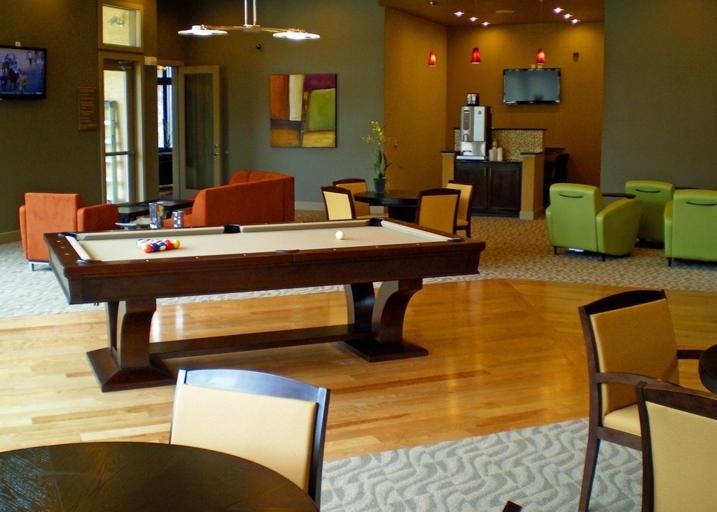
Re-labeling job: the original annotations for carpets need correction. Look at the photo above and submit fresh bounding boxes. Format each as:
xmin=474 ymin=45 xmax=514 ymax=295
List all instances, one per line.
xmin=1 ymin=208 xmax=714 ymax=320
xmin=319 ymin=415 xmax=644 ymax=512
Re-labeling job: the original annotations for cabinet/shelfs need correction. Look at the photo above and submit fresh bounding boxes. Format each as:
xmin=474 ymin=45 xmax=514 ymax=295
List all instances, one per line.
xmin=455 ymin=160 xmax=520 ymax=217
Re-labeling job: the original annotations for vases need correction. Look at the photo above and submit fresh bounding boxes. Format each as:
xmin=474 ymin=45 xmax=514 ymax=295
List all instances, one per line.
xmin=371 ymin=176 xmax=388 ymax=195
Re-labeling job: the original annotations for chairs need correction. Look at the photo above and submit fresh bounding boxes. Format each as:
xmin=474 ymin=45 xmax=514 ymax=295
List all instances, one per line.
xmin=634 ymin=380 xmax=716 ymax=510
xmin=574 ymin=285 xmax=707 ymax=510
xmin=414 ymin=185 xmax=461 ymax=238
xmin=334 ymin=177 xmax=371 ymax=217
xmin=445 ymin=178 xmax=477 ymax=240
xmin=165 ymin=362 xmax=333 ymax=509
xmin=319 ymin=182 xmax=363 ymax=220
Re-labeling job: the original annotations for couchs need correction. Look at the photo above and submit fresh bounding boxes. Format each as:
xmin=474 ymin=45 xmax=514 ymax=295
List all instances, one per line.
xmin=661 ymin=187 xmax=716 ymax=269
xmin=18 ymin=186 xmax=121 ymax=272
xmin=544 ymin=179 xmax=645 ymax=262
xmin=623 ymin=177 xmax=673 ymax=247
xmin=158 ymin=169 xmax=296 ymax=230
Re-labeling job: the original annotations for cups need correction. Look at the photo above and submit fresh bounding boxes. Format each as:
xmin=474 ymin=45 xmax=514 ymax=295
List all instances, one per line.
xmin=466 ymin=92 xmax=476 ymax=104
xmin=172 ymin=210 xmax=184 ymax=228
xmin=148 ymin=201 xmax=164 ymax=230
xmin=489 ymin=150 xmax=494 ymax=162
xmin=498 ymin=147 xmax=504 ymax=162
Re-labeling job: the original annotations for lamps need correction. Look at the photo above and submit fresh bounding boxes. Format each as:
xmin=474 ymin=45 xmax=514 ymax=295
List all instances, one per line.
xmin=174 ymin=0 xmax=321 ymax=43
xmin=425 ymin=1 xmax=437 ymax=66
xmin=534 ymin=45 xmax=545 ymax=65
xmin=470 ymin=47 xmax=480 ymax=65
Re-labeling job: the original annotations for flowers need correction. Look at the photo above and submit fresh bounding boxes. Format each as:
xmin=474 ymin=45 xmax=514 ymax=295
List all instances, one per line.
xmin=359 ymin=120 xmax=401 ymax=178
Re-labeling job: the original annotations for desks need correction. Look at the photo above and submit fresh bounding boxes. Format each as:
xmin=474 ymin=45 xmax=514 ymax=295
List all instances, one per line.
xmin=41 ymin=215 xmax=486 ymax=394
xmin=351 ymin=186 xmax=430 ymax=224
xmin=695 ymin=339 xmax=716 ymax=396
xmin=0 ymin=435 xmax=323 ymax=512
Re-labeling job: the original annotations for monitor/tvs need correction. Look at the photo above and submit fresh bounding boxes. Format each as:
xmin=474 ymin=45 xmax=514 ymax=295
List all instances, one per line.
xmin=0 ymin=45 xmax=47 ymax=101
xmin=503 ymin=67 xmax=560 ymax=104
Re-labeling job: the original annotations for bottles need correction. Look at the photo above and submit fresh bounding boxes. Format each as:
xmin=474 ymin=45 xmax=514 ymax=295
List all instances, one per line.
xmin=492 ymin=138 xmax=497 ymax=161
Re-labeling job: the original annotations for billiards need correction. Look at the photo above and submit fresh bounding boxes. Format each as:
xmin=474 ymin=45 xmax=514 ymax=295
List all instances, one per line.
xmin=335 ymin=231 xmax=344 ymax=240
xmin=136 ymin=238 xmax=180 ymax=253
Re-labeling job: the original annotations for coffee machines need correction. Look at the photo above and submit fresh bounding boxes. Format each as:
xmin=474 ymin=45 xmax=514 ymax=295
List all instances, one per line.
xmin=456 ymin=105 xmax=492 ymax=161
